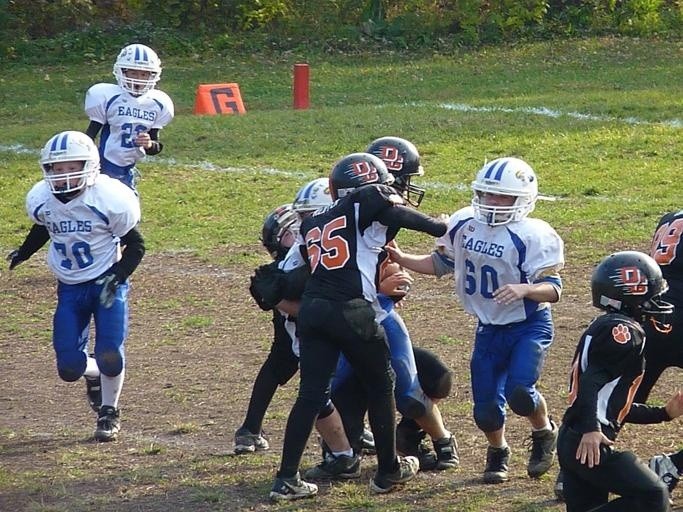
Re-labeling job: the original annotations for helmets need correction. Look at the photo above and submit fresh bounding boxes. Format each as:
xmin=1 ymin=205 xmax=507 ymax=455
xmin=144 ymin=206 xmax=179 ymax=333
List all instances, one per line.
xmin=366 ymin=135 xmax=426 ymax=179
xmin=329 ymin=154 xmax=395 ymax=194
xmin=471 ymin=156 xmax=538 ymax=226
xmin=261 ymin=203 xmax=298 ymax=255
xmin=294 ymin=177 xmax=332 ymax=212
xmin=592 ymin=249 xmax=675 ymax=324
xmin=39 ymin=130 xmax=100 ymax=196
xmin=113 ymin=42 xmax=159 ymax=97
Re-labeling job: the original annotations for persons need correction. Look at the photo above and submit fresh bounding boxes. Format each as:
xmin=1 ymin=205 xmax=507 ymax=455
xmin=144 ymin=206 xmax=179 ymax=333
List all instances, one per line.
xmin=613 ymin=209 xmax=683 ymax=443
xmin=559 ymin=251 xmax=682 ymax=508
xmin=6 ymin=131 xmax=146 ymax=442
xmin=384 ymin=157 xmax=562 ymax=481
xmin=84 ymin=44 xmax=174 ymax=185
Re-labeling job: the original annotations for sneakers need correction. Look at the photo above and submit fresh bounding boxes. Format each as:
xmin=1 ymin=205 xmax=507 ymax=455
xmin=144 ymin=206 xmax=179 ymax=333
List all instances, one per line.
xmin=527 ymin=420 xmax=559 ymax=477
xmin=83 ymin=354 xmax=102 ymax=410
xmin=233 ymin=434 xmax=271 ymax=452
xmin=370 ymin=455 xmax=420 ymax=491
xmin=432 ymin=435 xmax=461 ymax=472
xmin=94 ymin=403 xmax=122 ymax=442
xmin=647 ymin=454 xmax=680 ymax=499
xmin=302 ymin=455 xmax=361 ymax=478
xmin=270 ymin=480 xmax=319 ymax=500
xmin=482 ymin=445 xmax=512 ymax=482
xmin=396 ymin=426 xmax=437 ymax=470
xmin=357 ymin=428 xmax=380 ymax=455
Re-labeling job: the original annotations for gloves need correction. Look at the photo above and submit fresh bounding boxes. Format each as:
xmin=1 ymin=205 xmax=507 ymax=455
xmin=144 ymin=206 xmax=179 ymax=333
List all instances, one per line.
xmin=94 ymin=272 xmax=120 ymax=309
xmin=6 ymin=249 xmax=24 ymax=268
